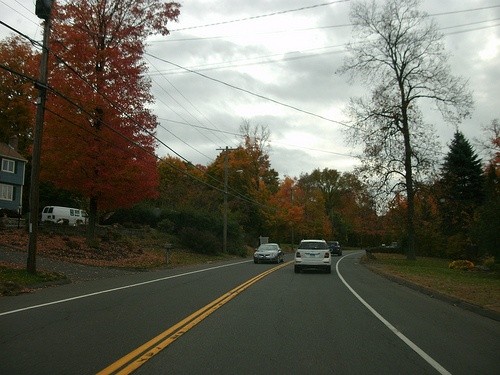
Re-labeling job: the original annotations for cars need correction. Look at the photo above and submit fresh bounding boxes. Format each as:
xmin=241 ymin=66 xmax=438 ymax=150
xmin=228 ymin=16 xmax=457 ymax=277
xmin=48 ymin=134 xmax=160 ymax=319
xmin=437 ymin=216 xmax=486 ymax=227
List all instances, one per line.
xmin=293 ymin=239 xmax=332 ymax=273
xmin=253 ymin=243 xmax=284 ymax=264
xmin=327 ymin=241 xmax=342 ymax=256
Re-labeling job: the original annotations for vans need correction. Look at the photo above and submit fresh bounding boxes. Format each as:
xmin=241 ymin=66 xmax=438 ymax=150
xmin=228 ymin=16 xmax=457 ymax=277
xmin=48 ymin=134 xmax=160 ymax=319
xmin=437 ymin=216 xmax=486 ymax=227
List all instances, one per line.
xmin=41 ymin=206 xmax=89 ymax=226
